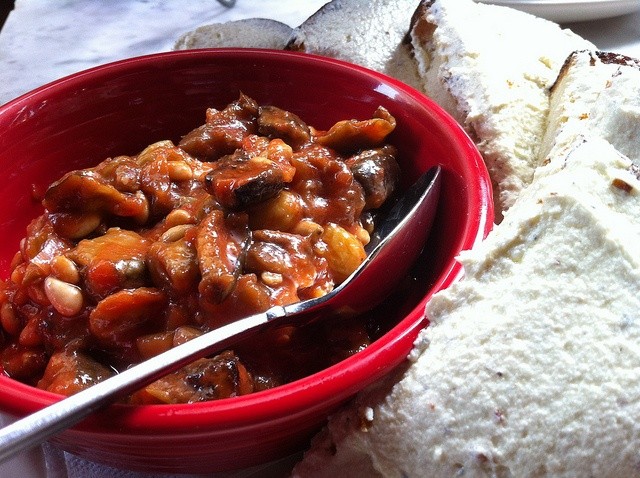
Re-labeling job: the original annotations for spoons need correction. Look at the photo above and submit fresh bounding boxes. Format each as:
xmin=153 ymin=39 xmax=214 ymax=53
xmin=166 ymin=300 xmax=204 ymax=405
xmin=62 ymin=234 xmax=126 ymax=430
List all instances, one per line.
xmin=1 ymin=165 xmax=439 ymax=460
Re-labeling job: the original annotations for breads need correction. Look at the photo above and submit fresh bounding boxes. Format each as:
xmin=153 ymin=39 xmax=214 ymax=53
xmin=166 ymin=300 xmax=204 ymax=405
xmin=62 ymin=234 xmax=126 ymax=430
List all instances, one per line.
xmin=170 ymin=0 xmax=640 ymax=478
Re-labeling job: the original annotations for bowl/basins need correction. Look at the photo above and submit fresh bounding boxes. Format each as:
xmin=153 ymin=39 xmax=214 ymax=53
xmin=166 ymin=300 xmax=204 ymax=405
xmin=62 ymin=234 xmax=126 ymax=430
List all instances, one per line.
xmin=0 ymin=48 xmax=497 ymax=466
